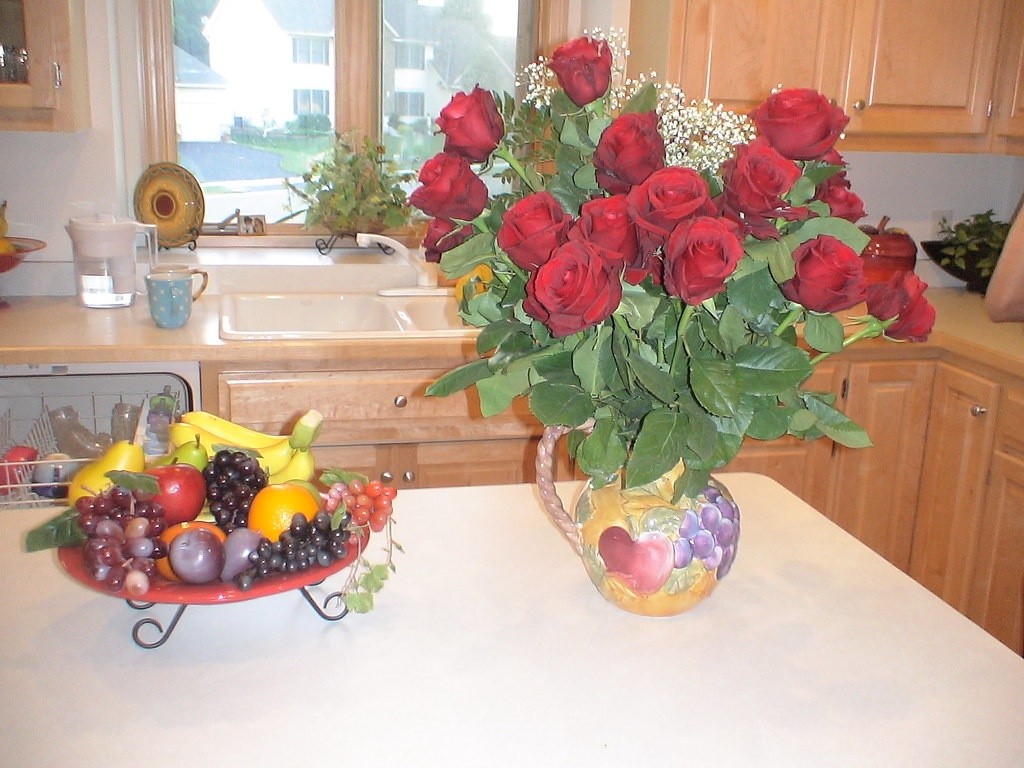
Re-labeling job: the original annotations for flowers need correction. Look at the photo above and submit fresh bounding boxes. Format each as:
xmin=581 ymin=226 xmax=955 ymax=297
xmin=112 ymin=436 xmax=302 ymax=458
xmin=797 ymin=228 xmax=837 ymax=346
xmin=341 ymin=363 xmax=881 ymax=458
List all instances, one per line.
xmin=295 ymin=131 xmax=417 ymax=230
xmin=410 ymin=27 xmax=934 ymax=507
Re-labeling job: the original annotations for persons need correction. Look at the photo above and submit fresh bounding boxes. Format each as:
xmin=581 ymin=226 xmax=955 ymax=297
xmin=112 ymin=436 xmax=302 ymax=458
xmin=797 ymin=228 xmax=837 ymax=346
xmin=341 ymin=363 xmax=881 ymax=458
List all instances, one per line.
xmin=985 ymin=203 xmax=1024 ymax=323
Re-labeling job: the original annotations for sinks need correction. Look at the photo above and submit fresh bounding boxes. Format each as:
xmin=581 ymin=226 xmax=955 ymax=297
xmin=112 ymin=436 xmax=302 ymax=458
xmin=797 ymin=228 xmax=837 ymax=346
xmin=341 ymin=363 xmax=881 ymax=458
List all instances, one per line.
xmin=219 ymin=290 xmax=402 ymax=341
xmin=403 ymin=295 xmax=485 ymax=339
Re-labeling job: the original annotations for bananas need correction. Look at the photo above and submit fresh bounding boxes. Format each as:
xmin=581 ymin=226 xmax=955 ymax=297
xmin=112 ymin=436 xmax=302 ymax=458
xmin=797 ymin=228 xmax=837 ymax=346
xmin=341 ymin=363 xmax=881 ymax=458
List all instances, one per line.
xmin=165 ymin=407 xmax=322 ymax=486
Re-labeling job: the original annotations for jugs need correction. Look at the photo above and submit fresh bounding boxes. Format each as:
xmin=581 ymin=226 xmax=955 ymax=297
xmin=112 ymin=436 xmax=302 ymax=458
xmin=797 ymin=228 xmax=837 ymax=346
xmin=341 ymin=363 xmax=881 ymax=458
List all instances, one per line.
xmin=64 ymin=213 xmax=158 ymax=308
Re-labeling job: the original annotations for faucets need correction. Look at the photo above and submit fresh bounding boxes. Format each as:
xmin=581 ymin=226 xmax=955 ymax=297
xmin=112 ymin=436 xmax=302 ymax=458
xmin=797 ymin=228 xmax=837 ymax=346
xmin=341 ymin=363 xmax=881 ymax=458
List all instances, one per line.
xmin=356 ymin=233 xmax=438 ymax=290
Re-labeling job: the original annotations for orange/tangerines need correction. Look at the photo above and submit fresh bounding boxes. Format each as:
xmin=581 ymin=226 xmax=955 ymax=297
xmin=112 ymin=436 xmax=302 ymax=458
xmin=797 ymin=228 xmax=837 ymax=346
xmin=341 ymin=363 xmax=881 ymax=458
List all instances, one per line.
xmin=247 ymin=483 xmax=318 ymax=542
xmin=157 ymin=520 xmax=225 ymax=580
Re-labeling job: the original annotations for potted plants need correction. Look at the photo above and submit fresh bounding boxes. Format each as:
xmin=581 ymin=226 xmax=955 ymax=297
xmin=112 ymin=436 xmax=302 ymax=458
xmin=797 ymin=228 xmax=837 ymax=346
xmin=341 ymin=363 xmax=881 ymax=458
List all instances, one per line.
xmin=921 ymin=210 xmax=1011 ymax=293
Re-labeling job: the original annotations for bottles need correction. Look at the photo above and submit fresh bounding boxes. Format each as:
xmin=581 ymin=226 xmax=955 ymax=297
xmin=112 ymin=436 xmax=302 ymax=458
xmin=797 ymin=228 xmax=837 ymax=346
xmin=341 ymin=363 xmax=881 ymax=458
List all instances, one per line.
xmin=149 ymin=385 xmax=175 ymax=413
xmin=144 ymin=400 xmax=171 ymax=453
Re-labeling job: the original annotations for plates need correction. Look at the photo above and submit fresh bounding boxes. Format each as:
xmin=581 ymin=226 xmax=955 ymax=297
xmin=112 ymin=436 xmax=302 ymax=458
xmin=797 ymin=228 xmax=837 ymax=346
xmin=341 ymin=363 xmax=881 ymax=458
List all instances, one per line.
xmin=133 ymin=162 xmax=204 ymax=248
xmin=57 ymin=489 xmax=369 ymax=606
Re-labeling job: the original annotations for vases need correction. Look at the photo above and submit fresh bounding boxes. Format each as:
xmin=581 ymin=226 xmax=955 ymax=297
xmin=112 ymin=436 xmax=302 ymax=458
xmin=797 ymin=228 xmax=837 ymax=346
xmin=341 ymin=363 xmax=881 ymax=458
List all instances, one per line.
xmin=534 ymin=417 xmax=739 ymax=618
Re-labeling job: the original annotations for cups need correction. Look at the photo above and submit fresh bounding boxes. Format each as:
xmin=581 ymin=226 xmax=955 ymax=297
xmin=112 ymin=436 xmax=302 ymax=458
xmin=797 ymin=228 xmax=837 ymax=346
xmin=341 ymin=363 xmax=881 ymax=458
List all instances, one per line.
xmin=144 ymin=272 xmax=194 ymax=328
xmin=49 ymin=403 xmax=141 ymax=464
xmin=149 ymin=263 xmax=208 ymax=302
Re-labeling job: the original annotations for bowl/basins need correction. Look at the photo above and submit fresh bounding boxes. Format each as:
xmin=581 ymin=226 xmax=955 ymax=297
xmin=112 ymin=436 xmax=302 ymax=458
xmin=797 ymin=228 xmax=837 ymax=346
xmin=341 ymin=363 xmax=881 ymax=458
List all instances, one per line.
xmin=32 ymin=452 xmax=78 ymax=497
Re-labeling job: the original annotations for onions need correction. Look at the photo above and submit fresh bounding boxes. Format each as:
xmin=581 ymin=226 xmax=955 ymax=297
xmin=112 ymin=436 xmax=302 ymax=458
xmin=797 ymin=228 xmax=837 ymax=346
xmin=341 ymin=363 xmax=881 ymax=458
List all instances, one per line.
xmin=168 ymin=526 xmax=266 ymax=585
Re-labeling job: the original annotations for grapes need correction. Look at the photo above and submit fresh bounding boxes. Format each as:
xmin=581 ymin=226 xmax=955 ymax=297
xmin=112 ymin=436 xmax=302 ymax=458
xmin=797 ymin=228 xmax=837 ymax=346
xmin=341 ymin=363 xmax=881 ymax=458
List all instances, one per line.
xmin=74 ymin=482 xmax=167 ymax=597
xmin=326 ymin=479 xmax=398 ymax=544
xmin=233 ymin=509 xmax=352 ymax=590
xmin=200 ymin=449 xmax=269 ymax=532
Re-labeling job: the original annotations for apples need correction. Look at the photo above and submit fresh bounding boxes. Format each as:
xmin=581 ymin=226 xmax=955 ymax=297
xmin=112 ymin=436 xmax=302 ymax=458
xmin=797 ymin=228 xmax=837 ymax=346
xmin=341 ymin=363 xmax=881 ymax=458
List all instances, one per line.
xmin=143 ymin=456 xmax=207 ymax=525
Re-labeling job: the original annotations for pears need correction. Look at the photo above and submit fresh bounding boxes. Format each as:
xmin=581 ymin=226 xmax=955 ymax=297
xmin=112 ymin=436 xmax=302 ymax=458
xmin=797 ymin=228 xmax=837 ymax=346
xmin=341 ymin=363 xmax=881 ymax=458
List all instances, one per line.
xmin=67 ymin=433 xmax=206 ymax=510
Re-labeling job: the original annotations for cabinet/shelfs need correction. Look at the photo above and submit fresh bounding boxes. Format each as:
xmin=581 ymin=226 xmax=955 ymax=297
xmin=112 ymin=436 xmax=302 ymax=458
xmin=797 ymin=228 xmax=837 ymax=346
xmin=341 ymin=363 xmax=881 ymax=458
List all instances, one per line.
xmin=0 ymin=288 xmax=1024 ymax=656
xmin=622 ymin=1 xmax=1024 ymax=158
